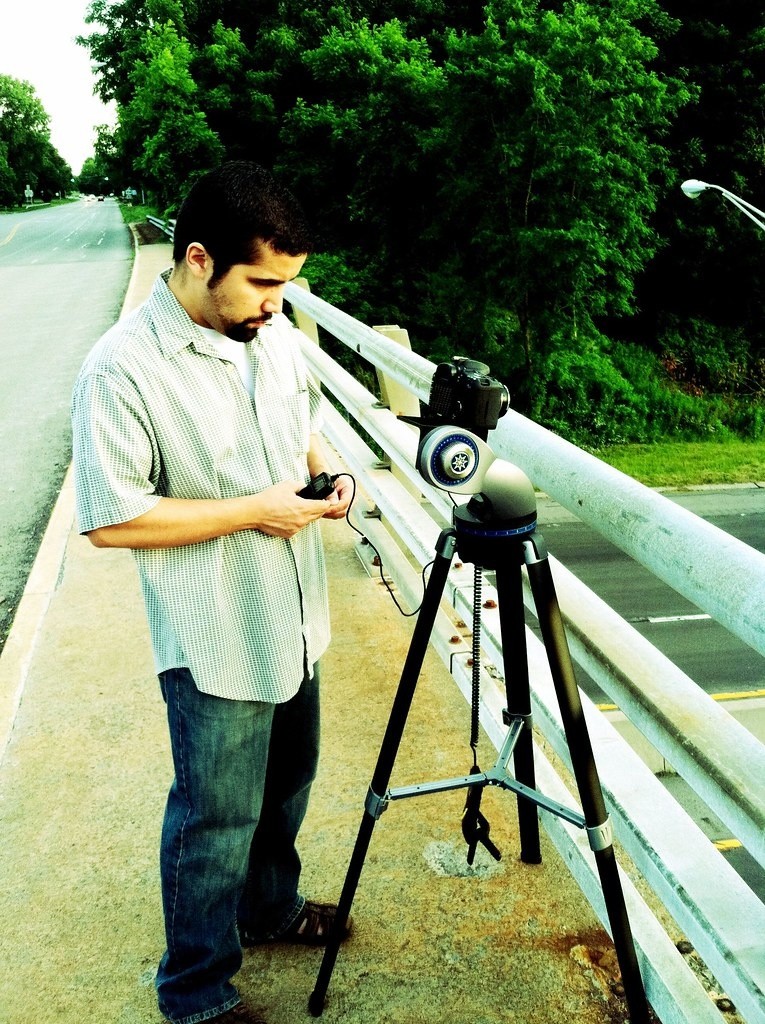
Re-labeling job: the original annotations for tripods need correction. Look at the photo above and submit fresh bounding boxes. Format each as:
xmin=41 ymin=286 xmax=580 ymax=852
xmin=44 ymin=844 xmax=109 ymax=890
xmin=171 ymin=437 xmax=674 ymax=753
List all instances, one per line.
xmin=308 ymin=416 xmax=651 ymax=1021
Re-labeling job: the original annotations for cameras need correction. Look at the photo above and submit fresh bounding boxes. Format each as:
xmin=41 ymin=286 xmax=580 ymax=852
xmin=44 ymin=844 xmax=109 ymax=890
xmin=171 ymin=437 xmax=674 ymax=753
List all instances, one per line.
xmin=427 ymin=356 xmax=510 ymax=430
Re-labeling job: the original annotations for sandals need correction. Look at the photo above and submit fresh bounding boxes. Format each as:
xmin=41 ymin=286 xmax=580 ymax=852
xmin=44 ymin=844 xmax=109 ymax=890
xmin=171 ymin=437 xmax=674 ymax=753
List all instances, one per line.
xmin=240 ymin=899 xmax=352 ymax=949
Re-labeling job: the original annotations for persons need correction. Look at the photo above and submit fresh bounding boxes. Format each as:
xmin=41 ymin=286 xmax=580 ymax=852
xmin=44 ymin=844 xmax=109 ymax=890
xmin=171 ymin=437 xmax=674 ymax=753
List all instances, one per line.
xmin=69 ymin=152 xmax=358 ymax=1024
